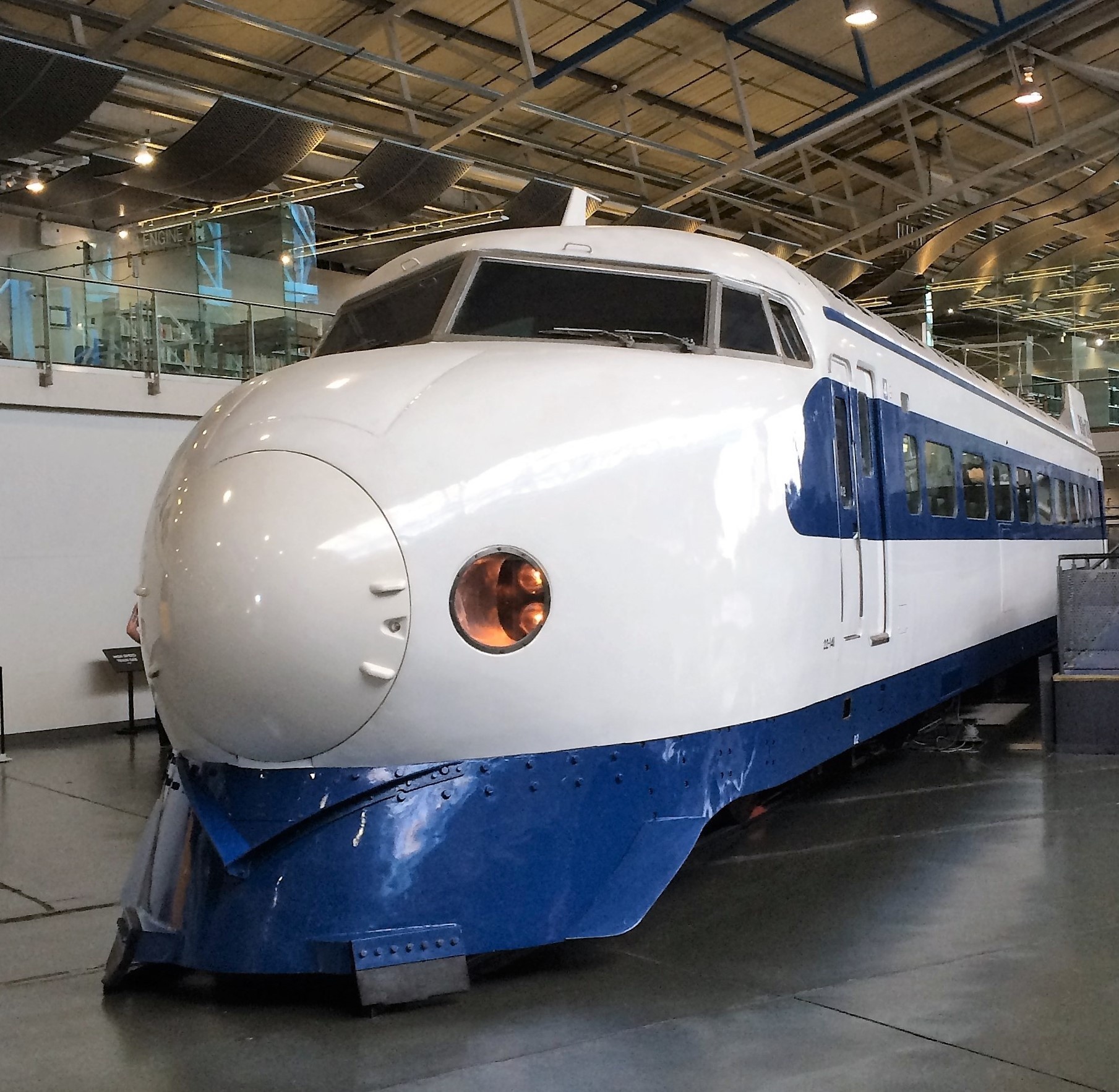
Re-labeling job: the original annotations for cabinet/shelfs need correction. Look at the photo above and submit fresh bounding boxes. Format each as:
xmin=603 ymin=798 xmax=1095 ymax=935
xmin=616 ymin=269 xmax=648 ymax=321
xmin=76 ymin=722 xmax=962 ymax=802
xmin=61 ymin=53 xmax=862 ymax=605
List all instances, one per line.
xmin=103 ymin=309 xmax=321 ymax=379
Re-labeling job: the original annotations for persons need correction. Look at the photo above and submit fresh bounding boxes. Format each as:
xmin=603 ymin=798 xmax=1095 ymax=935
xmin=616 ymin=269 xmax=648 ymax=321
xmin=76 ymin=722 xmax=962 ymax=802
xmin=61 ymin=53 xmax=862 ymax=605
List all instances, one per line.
xmin=127 ymin=603 xmax=172 ymax=750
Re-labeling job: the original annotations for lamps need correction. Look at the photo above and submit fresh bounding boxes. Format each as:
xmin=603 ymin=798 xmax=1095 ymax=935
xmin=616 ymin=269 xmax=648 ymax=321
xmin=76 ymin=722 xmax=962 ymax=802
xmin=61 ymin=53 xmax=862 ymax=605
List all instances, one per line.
xmin=0 ymin=166 xmax=45 ymax=191
xmin=121 ymin=178 xmax=510 ymax=263
xmin=1015 ymin=54 xmax=1043 ymax=103
xmin=133 ymin=137 xmax=154 ymax=164
xmin=845 ymin=0 xmax=878 ymax=24
xmin=854 ymin=258 xmax=1119 ymax=345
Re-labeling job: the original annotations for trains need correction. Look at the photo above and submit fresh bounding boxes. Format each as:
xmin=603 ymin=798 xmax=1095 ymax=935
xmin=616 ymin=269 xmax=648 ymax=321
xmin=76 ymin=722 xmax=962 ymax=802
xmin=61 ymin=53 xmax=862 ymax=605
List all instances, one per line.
xmin=98 ymin=220 xmax=1114 ymax=1018
